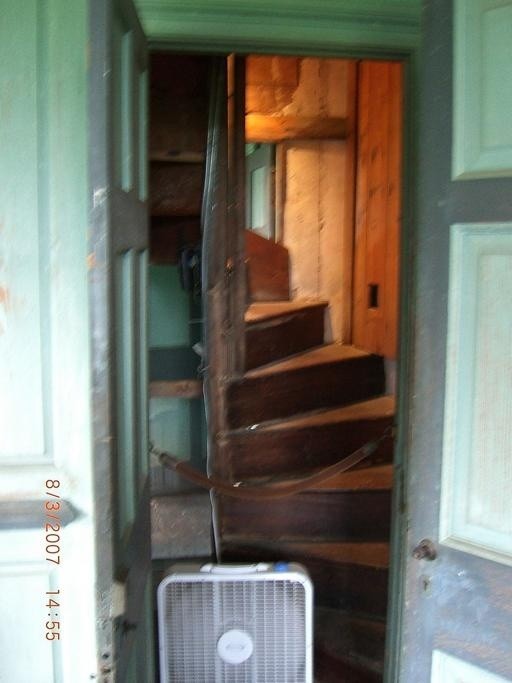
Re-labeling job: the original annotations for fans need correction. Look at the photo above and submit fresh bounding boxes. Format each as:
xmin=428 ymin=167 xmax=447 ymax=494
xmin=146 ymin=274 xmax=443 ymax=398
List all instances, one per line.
xmin=159 ymin=560 xmax=314 ymax=682
